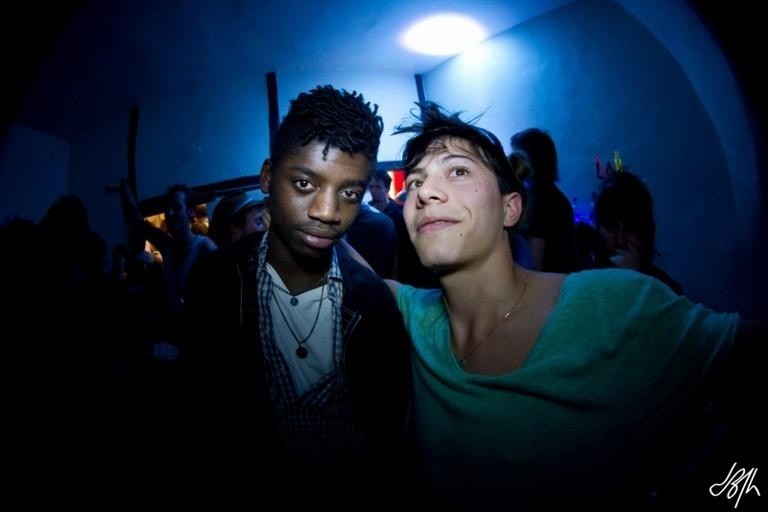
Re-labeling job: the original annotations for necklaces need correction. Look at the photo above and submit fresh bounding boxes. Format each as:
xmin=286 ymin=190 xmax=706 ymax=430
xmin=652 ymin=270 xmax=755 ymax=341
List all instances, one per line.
xmin=268 ymin=258 xmax=323 ymax=357
xmin=454 ymin=263 xmax=529 ymax=367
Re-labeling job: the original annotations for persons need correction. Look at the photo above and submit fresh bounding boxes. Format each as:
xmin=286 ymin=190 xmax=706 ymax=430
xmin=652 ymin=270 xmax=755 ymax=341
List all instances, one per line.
xmin=185 ymin=87 xmax=410 ymax=512
xmin=1 ymin=182 xmax=264 ymax=297
xmin=506 ymin=129 xmax=682 ymax=294
xmin=352 ymin=162 xmax=435 ymax=293
xmin=368 ymin=105 xmax=762 ymax=510
